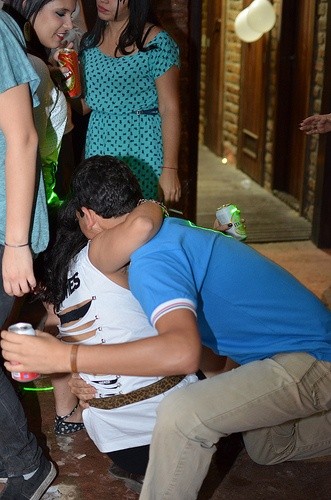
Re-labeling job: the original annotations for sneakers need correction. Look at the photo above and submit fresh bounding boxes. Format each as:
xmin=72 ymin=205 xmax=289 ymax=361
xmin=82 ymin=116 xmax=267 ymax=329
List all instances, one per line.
xmin=0 ymin=458 xmax=57 ymax=500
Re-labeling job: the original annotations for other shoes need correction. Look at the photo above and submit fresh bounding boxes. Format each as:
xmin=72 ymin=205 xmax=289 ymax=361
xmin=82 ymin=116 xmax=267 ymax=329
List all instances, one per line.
xmin=108 ymin=464 xmax=144 ymax=494
xmin=52 ymin=399 xmax=86 ymax=436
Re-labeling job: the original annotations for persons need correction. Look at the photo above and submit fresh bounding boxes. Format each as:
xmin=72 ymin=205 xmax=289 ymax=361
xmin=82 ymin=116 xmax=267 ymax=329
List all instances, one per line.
xmin=0 ymin=0 xmax=182 ymax=500
xmin=0 ymin=114 xmax=331 ymax=500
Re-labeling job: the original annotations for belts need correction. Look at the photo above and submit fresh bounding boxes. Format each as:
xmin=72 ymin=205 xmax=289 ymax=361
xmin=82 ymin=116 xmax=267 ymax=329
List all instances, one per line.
xmin=83 ymin=373 xmax=188 ymax=410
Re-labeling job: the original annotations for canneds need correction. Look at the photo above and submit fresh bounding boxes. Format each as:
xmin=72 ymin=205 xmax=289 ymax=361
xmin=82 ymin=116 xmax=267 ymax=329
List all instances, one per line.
xmin=8 ymin=323 xmax=40 ymax=382
xmin=58 ymin=48 xmax=82 ymax=99
xmin=215 ymin=203 xmax=248 ymax=241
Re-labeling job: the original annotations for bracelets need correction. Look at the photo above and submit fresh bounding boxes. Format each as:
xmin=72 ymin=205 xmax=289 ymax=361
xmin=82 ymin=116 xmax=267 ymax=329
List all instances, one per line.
xmin=70 ymin=344 xmax=80 ymax=372
xmin=162 ymin=167 xmax=178 ymax=169
xmin=4 ymin=242 xmax=31 ymax=248
